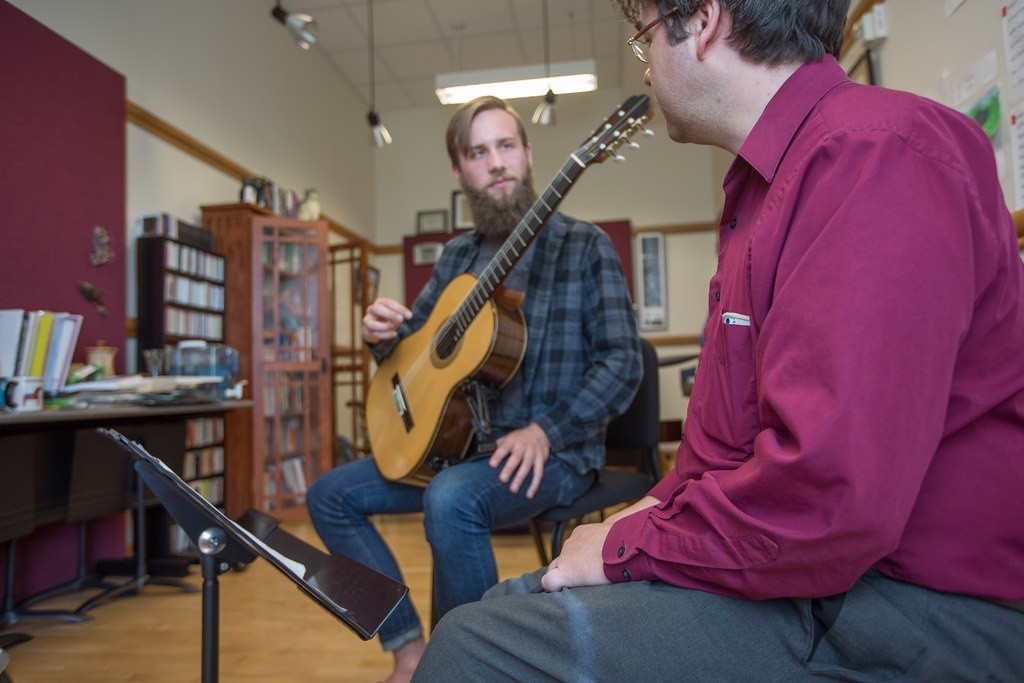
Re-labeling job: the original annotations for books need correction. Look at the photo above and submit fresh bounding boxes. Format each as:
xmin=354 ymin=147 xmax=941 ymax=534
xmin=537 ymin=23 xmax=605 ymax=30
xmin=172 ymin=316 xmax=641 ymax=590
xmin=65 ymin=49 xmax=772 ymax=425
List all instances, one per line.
xmin=263 ymin=243 xmax=313 ymax=509
xmin=0 ymin=309 xmax=224 ymax=405
xmin=164 ymin=242 xmax=225 ymax=551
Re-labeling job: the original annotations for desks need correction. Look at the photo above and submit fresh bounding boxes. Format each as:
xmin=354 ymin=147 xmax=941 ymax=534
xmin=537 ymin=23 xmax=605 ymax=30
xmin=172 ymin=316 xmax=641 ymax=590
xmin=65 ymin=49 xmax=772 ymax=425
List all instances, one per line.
xmin=0 ymin=398 xmax=252 ymax=652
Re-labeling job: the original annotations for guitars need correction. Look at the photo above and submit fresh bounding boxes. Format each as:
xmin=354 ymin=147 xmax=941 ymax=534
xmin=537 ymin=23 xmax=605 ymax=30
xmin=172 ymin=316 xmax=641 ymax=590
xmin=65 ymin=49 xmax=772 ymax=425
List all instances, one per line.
xmin=363 ymin=92 xmax=657 ymax=490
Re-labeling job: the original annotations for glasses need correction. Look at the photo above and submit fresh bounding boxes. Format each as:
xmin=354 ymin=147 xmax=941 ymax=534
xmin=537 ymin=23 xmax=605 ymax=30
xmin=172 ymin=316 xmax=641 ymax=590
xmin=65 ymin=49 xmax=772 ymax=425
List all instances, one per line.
xmin=628 ymin=4 xmax=684 ymax=63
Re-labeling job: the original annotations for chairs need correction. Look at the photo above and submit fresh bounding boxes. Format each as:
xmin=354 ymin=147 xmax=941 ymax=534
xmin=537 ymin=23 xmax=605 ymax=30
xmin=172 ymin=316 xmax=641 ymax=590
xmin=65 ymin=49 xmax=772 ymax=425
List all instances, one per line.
xmin=76 ymin=425 xmax=200 ymax=615
xmin=0 ymin=432 xmax=94 ymax=627
xmin=487 ymin=338 xmax=671 ymax=568
xmin=21 ymin=428 xmax=138 ymax=608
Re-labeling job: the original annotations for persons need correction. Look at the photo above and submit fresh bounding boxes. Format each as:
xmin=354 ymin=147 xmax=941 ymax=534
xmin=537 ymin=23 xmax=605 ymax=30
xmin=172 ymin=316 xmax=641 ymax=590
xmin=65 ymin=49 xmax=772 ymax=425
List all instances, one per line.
xmin=409 ymin=0 xmax=1024 ymax=683
xmin=305 ymin=95 xmax=644 ymax=683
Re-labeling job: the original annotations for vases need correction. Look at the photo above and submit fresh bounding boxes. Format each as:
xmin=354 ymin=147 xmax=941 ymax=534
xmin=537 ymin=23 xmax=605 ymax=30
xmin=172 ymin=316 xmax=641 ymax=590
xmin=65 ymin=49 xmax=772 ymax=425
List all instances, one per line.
xmin=294 ymin=189 xmax=323 ymax=222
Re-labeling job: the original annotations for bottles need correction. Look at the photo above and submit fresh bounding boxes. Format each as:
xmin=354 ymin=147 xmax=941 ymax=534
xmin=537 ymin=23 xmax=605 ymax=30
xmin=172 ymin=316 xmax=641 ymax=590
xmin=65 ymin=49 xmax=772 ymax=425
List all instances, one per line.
xmin=239 ymin=173 xmax=322 ymax=222
xmin=84 ymin=345 xmax=118 ymax=380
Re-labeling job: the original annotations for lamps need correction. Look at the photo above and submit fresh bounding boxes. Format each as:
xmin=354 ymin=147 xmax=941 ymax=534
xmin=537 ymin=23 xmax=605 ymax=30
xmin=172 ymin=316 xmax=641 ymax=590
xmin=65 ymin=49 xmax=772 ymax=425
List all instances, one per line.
xmin=366 ymin=0 xmax=392 ymax=149
xmin=531 ymin=0 xmax=557 ymax=127
xmin=433 ymin=0 xmax=599 ymax=106
xmin=271 ymin=0 xmax=319 ymax=50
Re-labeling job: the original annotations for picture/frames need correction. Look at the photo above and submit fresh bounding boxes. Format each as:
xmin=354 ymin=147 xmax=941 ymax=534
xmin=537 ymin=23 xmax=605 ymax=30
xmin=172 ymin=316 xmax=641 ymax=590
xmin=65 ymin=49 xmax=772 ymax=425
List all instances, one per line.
xmin=354 ymin=266 xmax=380 ymax=305
xmin=416 ymin=208 xmax=448 ymax=233
xmin=451 ymin=190 xmax=475 ymax=230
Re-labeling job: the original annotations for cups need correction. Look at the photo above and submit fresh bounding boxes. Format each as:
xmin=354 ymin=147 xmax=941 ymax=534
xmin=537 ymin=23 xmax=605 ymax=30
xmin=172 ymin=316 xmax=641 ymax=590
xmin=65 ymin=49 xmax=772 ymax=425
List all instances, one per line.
xmin=144 ymin=345 xmax=238 ymax=401
xmin=4 ymin=375 xmax=45 ymax=411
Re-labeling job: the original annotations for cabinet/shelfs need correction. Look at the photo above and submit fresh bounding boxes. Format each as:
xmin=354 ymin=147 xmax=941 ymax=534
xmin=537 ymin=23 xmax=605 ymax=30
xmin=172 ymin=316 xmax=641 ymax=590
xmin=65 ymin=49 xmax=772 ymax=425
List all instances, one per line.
xmin=200 ymin=202 xmax=369 ymax=522
xmin=134 ymin=235 xmax=227 ymax=562
